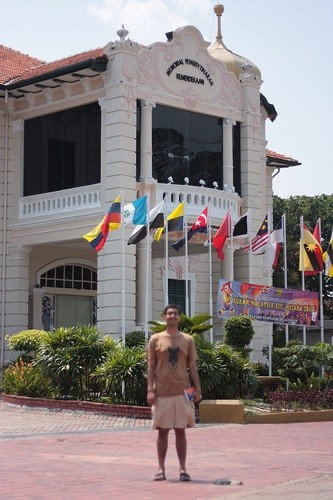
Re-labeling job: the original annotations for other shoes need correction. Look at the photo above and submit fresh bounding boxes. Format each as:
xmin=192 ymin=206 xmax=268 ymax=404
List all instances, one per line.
xmin=180 ymin=473 xmax=191 ymax=481
xmin=153 ymin=473 xmax=166 ymax=480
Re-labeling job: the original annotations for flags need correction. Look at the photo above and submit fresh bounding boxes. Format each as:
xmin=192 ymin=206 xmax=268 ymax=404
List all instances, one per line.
xmin=82 ymin=191 xmax=333 ymax=279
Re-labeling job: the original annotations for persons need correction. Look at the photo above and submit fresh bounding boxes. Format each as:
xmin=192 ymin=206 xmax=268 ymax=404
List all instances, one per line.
xmin=147 ymin=304 xmax=202 ymax=481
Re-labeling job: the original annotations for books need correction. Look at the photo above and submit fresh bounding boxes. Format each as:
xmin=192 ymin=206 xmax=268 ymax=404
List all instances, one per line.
xmin=184 ymin=386 xmax=197 ymax=402
